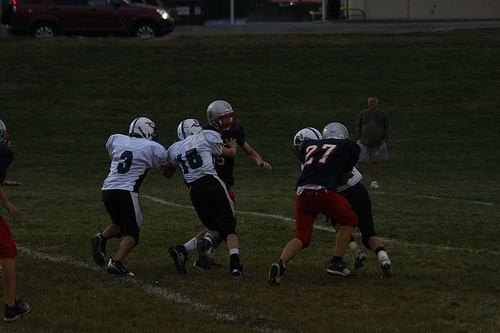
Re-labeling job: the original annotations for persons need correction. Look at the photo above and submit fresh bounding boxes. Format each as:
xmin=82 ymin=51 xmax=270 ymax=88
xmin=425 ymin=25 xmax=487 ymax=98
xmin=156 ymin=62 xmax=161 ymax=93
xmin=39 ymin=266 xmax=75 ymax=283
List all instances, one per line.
xmin=354 ymin=95 xmax=390 ymax=189
xmin=166 ymin=119 xmax=245 ymax=278
xmin=91 ymin=117 xmax=167 ymax=276
xmin=0 ymin=120 xmax=30 ymax=321
xmin=266 ymin=122 xmax=392 ymax=287
xmin=193 ymin=101 xmax=273 ymax=271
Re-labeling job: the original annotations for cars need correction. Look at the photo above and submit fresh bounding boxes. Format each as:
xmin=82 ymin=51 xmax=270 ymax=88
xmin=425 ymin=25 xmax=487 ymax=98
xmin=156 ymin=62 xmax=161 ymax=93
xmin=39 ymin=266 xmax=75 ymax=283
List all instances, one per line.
xmin=6 ymin=0 xmax=175 ymax=39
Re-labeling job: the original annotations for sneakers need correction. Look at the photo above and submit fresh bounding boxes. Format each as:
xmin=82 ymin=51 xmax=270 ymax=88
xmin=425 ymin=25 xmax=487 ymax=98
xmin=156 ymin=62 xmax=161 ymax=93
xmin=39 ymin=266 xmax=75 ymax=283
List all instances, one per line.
xmin=354 ymin=251 xmax=367 ymax=274
xmin=231 ymin=254 xmax=245 ymax=276
xmin=370 ymin=181 xmax=380 ymax=188
xmin=106 ymin=256 xmax=135 ymax=277
xmin=326 ymin=258 xmax=353 ymax=277
xmin=4 ymin=298 xmax=29 ymax=322
xmin=168 ymin=244 xmax=189 ymax=276
xmin=268 ymin=261 xmax=286 ymax=285
xmin=380 ymin=258 xmax=393 ymax=280
xmin=91 ymin=232 xmax=107 ymax=267
xmin=192 ymin=238 xmax=221 ymax=269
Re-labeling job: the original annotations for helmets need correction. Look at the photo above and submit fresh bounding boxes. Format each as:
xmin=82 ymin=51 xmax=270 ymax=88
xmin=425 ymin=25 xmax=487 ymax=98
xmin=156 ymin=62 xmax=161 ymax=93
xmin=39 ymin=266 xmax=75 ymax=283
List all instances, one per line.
xmin=177 ymin=118 xmax=199 ymax=141
xmin=293 ymin=126 xmax=322 ymax=151
xmin=206 ymin=99 xmax=235 ymax=130
xmin=323 ymin=122 xmax=349 ymax=140
xmin=0 ymin=120 xmax=10 ymax=144
xmin=129 ymin=116 xmax=159 ymax=140
